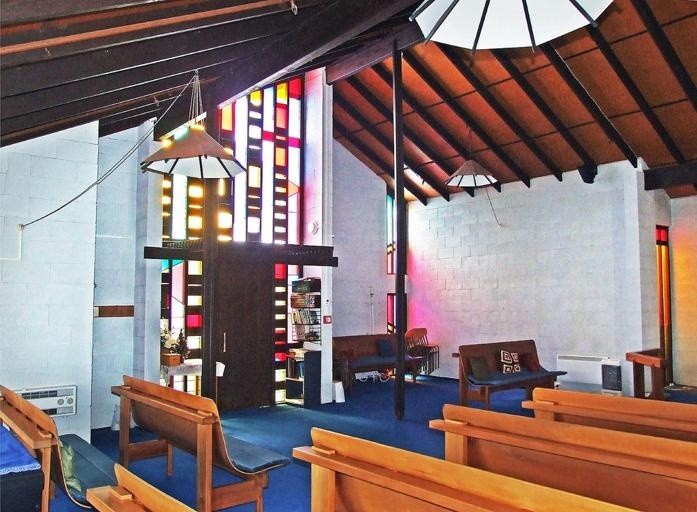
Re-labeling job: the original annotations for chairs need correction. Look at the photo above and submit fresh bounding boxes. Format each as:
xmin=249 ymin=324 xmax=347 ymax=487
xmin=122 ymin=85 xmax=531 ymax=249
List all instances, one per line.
xmin=407 ymin=328 xmax=440 ymax=374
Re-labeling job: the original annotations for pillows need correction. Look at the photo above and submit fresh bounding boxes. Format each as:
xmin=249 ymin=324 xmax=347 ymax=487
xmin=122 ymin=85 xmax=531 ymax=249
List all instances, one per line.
xmin=374 ymin=339 xmax=394 ymax=359
xmin=59 ymin=444 xmax=82 ymax=493
xmin=496 ymin=350 xmax=522 ymax=377
xmin=469 ymin=356 xmax=492 ymax=381
xmin=525 ymin=352 xmax=541 ymax=371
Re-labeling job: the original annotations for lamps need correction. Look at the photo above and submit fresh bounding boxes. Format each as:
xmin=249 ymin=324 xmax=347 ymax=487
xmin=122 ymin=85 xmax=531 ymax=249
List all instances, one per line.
xmin=441 ymin=128 xmax=499 ymax=187
xmin=408 ymin=0 xmax=614 ymax=56
xmin=139 ymin=69 xmax=248 ymax=180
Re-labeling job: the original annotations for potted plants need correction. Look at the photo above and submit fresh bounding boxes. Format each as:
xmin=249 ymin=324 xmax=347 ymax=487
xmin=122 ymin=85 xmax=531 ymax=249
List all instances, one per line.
xmin=161 ymin=329 xmax=181 ymax=367
xmin=170 ymin=327 xmax=191 ymax=363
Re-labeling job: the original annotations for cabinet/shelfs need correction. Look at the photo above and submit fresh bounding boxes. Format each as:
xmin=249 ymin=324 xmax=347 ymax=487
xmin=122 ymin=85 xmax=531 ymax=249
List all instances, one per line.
xmin=291 ymin=276 xmax=321 ymax=341
xmin=286 ymin=348 xmax=321 ymax=407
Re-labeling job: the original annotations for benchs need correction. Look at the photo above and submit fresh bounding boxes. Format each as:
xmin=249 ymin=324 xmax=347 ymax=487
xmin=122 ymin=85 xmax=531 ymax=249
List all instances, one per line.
xmin=288 ymin=425 xmax=634 ymax=512
xmin=452 ymin=339 xmax=567 ymax=410
xmin=522 ymin=389 xmax=697 ymax=442
xmin=333 ymin=333 xmax=424 ymax=392
xmin=111 ymin=374 xmax=291 ymax=512
xmin=86 ymin=463 xmax=199 ymax=511
xmin=0 ymin=384 xmax=125 ymax=512
xmin=425 ymin=404 xmax=696 ymax=511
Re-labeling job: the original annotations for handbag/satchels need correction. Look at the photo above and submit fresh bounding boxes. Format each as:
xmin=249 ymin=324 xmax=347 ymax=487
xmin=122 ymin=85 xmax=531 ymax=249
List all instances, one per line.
xmin=376 ymin=339 xmax=394 ymax=357
